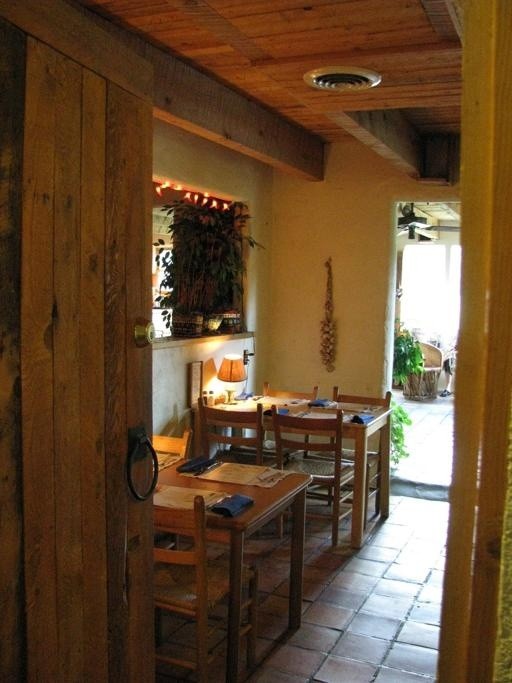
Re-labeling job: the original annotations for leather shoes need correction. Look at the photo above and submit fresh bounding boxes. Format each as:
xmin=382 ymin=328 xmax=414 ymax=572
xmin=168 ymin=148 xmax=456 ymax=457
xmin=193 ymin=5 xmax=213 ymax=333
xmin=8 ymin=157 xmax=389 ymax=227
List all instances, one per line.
xmin=440 ymin=389 xmax=452 ymax=397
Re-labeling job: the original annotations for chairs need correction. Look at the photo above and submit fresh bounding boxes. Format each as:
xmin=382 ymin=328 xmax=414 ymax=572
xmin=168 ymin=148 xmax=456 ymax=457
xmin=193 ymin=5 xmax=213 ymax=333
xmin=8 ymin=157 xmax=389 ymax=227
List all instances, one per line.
xmin=306 ymin=386 xmax=393 ymax=516
xmin=271 ymin=403 xmax=358 ymax=548
xmin=400 ymin=340 xmax=444 ymax=401
xmin=151 ymin=430 xmax=195 ymax=567
xmin=235 ymin=383 xmax=319 ymax=459
xmin=152 ymin=495 xmax=261 ymax=683
xmin=197 ymin=396 xmax=282 ymax=474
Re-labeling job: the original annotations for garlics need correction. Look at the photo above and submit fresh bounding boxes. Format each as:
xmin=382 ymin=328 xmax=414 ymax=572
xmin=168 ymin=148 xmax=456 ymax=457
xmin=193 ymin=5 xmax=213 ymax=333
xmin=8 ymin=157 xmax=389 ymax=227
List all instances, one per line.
xmin=320 ymin=319 xmax=336 ymax=365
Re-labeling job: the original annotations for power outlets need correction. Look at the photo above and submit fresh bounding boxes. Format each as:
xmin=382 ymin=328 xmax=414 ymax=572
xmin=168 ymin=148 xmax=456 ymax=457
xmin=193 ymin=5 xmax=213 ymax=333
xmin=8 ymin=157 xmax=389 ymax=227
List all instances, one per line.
xmin=243 ymin=349 xmax=250 ymax=365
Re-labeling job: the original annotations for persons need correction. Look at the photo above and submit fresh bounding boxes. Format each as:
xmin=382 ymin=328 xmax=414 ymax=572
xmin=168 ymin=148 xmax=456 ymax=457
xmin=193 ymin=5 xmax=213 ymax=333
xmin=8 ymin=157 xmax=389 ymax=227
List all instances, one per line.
xmin=440 ymin=346 xmax=457 ymax=398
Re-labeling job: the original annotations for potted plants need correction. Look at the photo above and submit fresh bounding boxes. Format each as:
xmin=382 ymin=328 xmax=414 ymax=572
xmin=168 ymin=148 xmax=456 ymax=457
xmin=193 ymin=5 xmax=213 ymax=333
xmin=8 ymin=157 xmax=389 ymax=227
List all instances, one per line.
xmin=154 ymin=193 xmax=268 ymax=338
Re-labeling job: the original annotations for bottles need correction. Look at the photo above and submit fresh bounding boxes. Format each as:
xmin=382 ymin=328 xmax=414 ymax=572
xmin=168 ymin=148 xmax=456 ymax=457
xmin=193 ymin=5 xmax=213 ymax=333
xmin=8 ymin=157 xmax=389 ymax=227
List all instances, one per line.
xmin=208 ymin=391 xmax=215 ymax=406
xmin=203 ymin=391 xmax=207 ymax=404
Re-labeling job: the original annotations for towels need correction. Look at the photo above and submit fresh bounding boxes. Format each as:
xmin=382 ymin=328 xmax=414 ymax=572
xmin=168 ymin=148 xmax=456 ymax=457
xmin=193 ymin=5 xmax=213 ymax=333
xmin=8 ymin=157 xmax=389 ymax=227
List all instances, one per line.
xmin=351 ymin=412 xmax=376 ymax=428
xmin=234 ymin=390 xmax=256 ymax=402
xmin=308 ymin=398 xmax=334 ymax=409
xmin=262 ymin=407 xmax=292 ymax=421
xmin=175 ymin=454 xmax=215 ymax=472
xmin=209 ymin=492 xmax=254 ymax=520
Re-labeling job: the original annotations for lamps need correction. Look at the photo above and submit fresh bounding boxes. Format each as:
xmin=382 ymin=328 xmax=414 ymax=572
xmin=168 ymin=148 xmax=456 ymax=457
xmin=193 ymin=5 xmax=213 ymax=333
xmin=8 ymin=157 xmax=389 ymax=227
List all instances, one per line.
xmin=217 ymin=353 xmax=249 ymax=406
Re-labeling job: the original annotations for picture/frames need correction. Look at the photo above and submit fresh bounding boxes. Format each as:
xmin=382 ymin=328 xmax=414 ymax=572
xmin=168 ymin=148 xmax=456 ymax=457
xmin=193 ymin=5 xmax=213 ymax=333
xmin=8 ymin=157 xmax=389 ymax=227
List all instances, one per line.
xmin=187 ymin=360 xmax=205 ymax=407
